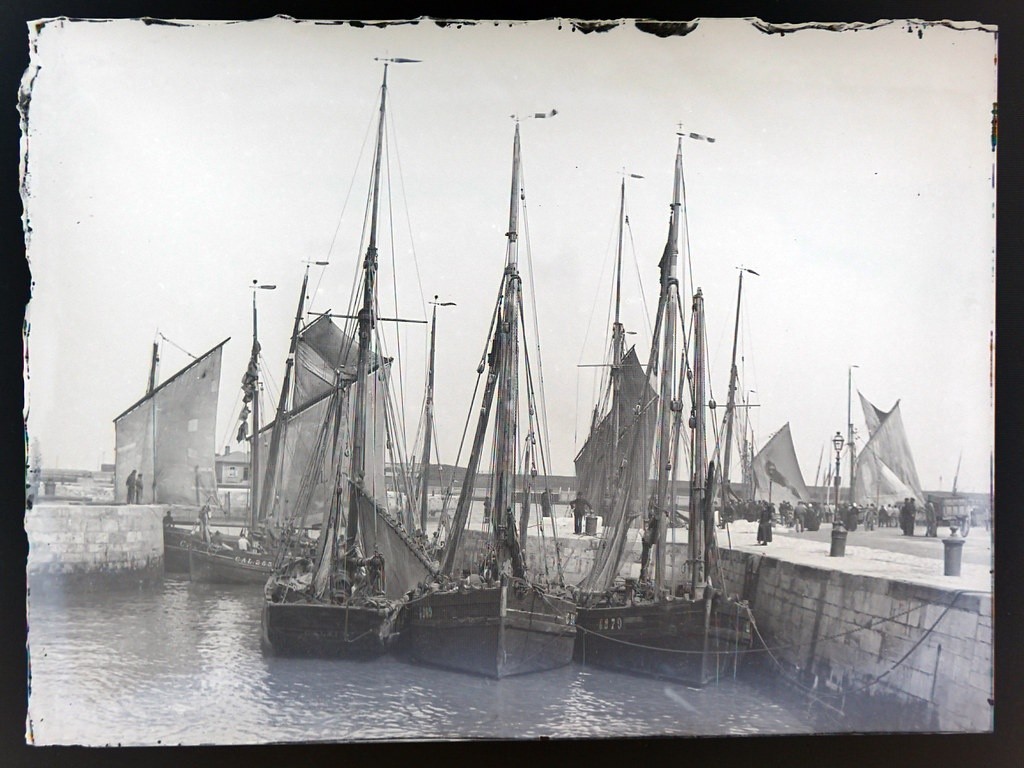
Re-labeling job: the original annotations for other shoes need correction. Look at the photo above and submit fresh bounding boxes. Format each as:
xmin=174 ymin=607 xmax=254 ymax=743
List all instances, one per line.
xmin=797 ymin=530 xmax=799 ymax=532
xmin=762 ymin=543 xmax=767 ymax=545
xmin=801 ymin=530 xmax=804 ymax=532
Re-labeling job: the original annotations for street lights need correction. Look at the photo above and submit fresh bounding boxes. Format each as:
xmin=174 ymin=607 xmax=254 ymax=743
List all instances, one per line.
xmin=833 ymin=430 xmax=845 ymax=526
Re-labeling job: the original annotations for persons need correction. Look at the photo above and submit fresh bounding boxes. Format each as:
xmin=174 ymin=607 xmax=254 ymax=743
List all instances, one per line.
xmin=569 ymin=492 xmax=594 ymax=536
xmin=125 ymin=470 xmax=144 ymax=504
xmin=736 ymin=497 xmax=917 ymax=546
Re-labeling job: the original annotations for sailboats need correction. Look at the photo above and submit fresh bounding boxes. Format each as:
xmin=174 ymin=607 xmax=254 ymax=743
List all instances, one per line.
xmin=160 ymin=53 xmax=768 ymax=685
